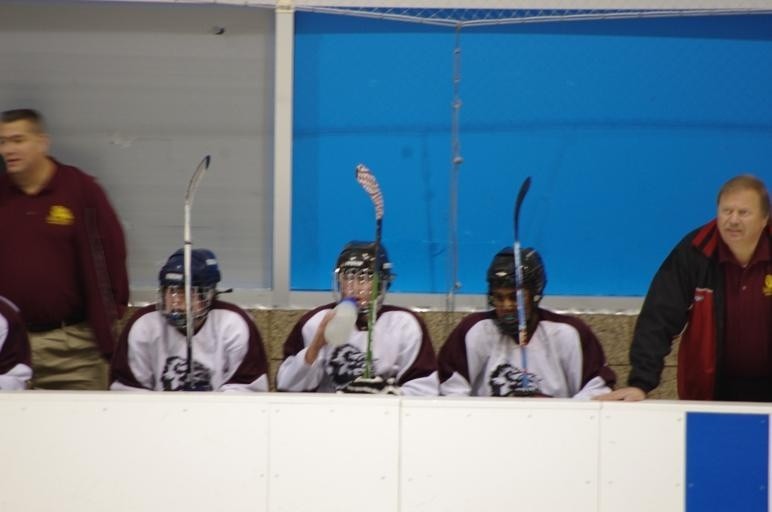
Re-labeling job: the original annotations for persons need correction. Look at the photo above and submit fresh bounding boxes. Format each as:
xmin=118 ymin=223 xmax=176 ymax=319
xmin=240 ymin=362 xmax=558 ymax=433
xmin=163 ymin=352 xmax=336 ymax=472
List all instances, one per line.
xmin=438 ymin=245 xmax=617 ymax=403
xmin=592 ymin=176 xmax=772 ymax=401
xmin=271 ymin=241 xmax=436 ymax=397
xmin=0 ymin=295 xmax=34 ymax=392
xmin=1 ymin=109 xmax=129 ymax=393
xmin=108 ymin=248 xmax=268 ymax=392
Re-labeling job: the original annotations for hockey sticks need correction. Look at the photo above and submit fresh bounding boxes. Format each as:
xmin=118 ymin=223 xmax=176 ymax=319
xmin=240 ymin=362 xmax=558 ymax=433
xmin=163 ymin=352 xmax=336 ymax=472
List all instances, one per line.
xmin=180 ymin=155 xmax=210 ymax=391
xmin=513 ymin=176 xmax=534 ymax=391
xmin=355 ymin=164 xmax=384 ymax=382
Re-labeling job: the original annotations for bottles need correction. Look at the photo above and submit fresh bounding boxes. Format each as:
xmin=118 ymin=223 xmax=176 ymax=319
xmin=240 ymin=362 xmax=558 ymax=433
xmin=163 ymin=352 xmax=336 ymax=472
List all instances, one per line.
xmin=323 ymin=295 xmax=358 ymax=349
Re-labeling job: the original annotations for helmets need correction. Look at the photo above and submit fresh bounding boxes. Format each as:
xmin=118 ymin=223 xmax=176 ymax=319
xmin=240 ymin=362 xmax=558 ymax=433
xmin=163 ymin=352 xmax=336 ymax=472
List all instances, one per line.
xmin=486 ymin=246 xmax=546 ymax=333
xmin=331 ymin=240 xmax=396 ymax=314
xmin=156 ymin=248 xmax=221 ymax=328
